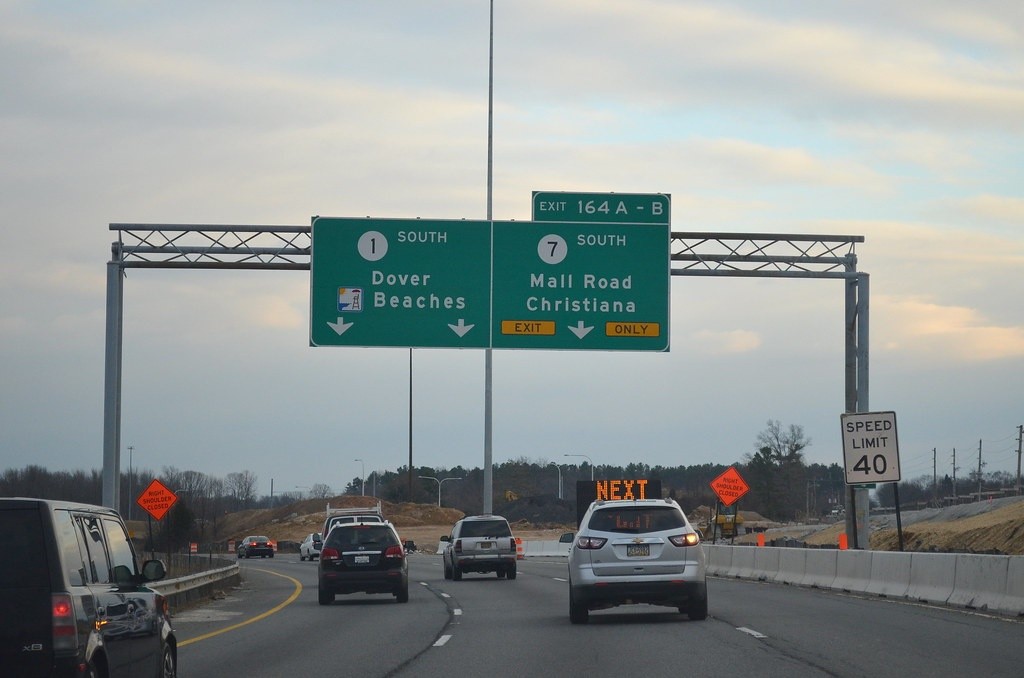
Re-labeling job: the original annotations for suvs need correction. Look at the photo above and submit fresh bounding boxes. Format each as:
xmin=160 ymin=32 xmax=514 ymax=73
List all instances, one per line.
xmin=0 ymin=496 xmax=179 ymax=678
xmin=440 ymin=514 xmax=518 ymax=582
xmin=311 ymin=500 xmax=416 ymax=605
xmin=559 ymin=496 xmax=709 ymax=626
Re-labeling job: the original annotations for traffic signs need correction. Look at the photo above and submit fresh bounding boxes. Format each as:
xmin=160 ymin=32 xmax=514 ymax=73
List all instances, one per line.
xmin=307 ymin=214 xmax=492 ymax=350
xmin=490 ymin=219 xmax=672 ymax=354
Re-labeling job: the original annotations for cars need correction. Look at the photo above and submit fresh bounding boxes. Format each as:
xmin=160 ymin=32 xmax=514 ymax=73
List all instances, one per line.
xmin=299 ymin=532 xmax=323 ymax=561
xmin=237 ymin=535 xmax=275 ymax=559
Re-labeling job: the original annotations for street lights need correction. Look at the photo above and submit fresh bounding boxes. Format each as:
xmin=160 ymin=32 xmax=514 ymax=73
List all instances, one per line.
xmin=418 ymin=475 xmax=462 ymax=507
xmin=564 ymin=454 xmax=594 ymax=481
xmin=354 ymin=459 xmax=366 ymax=496
xmin=127 ymin=444 xmax=135 ymax=519
xmin=550 ymin=463 xmax=562 ymax=499
